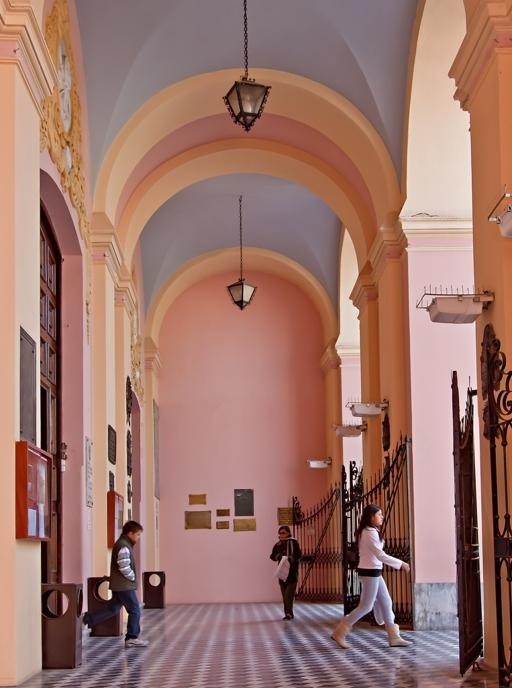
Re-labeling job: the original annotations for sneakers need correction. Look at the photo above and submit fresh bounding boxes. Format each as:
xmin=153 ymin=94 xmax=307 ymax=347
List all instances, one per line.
xmin=125 ymin=638 xmax=150 ymax=648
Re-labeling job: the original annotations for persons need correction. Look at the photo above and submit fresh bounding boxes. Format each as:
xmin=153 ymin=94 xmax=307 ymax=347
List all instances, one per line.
xmin=82 ymin=520 xmax=150 ymax=648
xmin=330 ymin=504 xmax=413 ymax=649
xmin=270 ymin=526 xmax=302 ymax=621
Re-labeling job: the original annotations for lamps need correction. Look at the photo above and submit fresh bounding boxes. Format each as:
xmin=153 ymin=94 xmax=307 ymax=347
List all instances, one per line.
xmin=222 ymin=0 xmax=272 ymax=134
xmin=227 ymin=195 xmax=258 ymax=311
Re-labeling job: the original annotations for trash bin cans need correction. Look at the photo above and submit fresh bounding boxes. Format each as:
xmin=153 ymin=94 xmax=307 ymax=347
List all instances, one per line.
xmin=42 ymin=583 xmax=83 ymax=668
xmin=88 ymin=578 xmax=122 ymax=636
xmin=142 ymin=571 xmax=165 ymax=608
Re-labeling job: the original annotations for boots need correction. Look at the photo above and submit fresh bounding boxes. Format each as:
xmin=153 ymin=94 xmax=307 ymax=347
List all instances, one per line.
xmin=331 ymin=618 xmax=352 ymax=649
xmin=386 ymin=624 xmax=410 ymax=646
